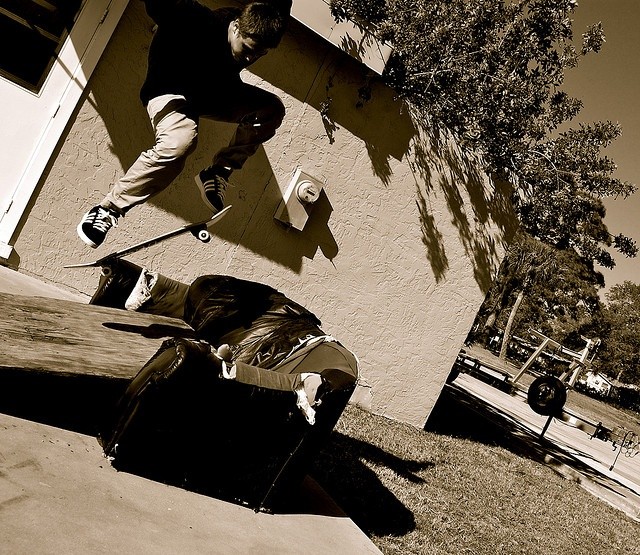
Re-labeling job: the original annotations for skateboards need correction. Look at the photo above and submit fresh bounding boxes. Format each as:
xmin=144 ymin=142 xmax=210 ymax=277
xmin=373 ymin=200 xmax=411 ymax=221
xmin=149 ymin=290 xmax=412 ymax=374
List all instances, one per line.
xmin=64 ymin=205 xmax=234 ymax=277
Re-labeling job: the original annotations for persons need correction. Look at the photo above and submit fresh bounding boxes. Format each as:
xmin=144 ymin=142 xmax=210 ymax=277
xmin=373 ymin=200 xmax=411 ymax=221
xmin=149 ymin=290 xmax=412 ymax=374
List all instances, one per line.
xmin=76 ymin=0 xmax=288 ymax=249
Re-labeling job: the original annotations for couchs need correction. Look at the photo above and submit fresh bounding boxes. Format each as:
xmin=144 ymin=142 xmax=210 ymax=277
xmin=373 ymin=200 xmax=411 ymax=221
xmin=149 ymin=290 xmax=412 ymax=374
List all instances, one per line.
xmin=88 ymin=258 xmax=360 ymax=517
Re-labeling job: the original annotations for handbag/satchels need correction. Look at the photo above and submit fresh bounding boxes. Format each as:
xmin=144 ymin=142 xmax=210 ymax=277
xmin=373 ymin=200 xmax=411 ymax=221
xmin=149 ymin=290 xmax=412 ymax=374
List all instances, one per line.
xmin=183 ymin=275 xmax=277 ymax=340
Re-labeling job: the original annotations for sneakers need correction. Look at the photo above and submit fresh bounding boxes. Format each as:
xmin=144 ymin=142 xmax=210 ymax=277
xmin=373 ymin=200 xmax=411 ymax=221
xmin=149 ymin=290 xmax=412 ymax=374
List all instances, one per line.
xmin=194 ymin=165 xmax=235 ymax=214
xmin=77 ymin=204 xmax=120 ymax=249
